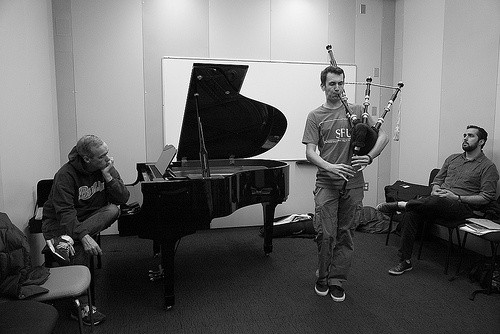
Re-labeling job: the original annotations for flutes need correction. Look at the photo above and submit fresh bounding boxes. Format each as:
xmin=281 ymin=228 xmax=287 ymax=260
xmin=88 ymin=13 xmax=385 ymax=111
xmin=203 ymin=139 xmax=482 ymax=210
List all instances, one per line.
xmin=325 ymin=44 xmax=405 ymax=197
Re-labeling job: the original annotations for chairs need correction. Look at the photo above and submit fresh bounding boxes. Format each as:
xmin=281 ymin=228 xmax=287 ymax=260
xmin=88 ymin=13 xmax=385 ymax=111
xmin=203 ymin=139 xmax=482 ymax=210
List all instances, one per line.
xmin=386 ymin=168 xmax=500 ymax=301
xmin=0 ymin=179 xmax=102 ymax=334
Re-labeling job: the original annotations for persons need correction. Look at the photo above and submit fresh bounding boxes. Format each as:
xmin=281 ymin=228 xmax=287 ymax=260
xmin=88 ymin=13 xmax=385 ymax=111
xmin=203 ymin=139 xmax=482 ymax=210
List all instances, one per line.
xmin=376 ymin=125 xmax=500 ymax=275
xmin=302 ymin=66 xmax=390 ymax=301
xmin=41 ymin=135 xmax=130 ymax=326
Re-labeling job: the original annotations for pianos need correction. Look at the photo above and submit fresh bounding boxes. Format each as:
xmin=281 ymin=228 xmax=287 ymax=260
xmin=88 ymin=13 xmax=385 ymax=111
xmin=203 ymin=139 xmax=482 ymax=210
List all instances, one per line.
xmin=117 ymin=63 xmax=290 ymax=310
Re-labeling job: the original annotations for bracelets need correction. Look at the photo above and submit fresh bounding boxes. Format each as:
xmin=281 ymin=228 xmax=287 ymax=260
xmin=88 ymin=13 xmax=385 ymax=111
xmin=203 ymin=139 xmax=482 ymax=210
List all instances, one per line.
xmin=456 ymin=193 xmax=462 ymax=203
xmin=366 ymin=154 xmax=372 ymax=165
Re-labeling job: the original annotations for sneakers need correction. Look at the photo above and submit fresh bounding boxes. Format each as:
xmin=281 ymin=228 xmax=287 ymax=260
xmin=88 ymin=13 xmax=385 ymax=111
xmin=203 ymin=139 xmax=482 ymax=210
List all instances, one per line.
xmin=378 ymin=201 xmax=404 ymax=212
xmin=47 ymin=237 xmax=75 ymax=260
xmin=71 ymin=305 xmax=105 ymax=325
xmin=315 ymin=281 xmax=329 ymax=296
xmin=388 ymin=259 xmax=412 ymax=274
xmin=329 ymin=285 xmax=346 ymax=302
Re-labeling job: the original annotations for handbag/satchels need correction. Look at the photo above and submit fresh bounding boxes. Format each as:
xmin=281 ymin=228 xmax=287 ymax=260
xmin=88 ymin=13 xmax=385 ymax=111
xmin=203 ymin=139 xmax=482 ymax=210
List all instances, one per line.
xmin=384 ymin=180 xmax=432 ymax=203
xmin=479 ymin=262 xmax=500 ymax=294
